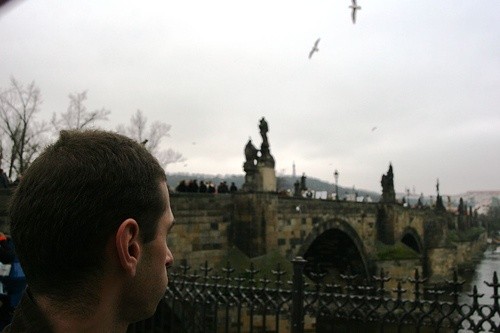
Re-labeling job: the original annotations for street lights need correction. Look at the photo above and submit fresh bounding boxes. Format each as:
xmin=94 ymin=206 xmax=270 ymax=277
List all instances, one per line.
xmin=7 ymin=122 xmax=21 ymax=178
xmin=334 ymin=169 xmax=339 ymax=201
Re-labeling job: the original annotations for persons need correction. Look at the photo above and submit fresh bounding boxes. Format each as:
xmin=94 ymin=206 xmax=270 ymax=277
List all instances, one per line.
xmin=175 ymin=179 xmax=238 ymax=193
xmin=1 ymin=129 xmax=175 ymax=333
xmin=0 ymin=229 xmax=26 ymax=331
xmin=242 ymin=117 xmax=269 ymax=173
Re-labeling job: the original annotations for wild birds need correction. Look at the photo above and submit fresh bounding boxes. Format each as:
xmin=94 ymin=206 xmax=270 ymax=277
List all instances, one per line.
xmin=348 ymin=0 xmax=362 ymax=25
xmin=308 ymin=38 xmax=320 ymax=59
